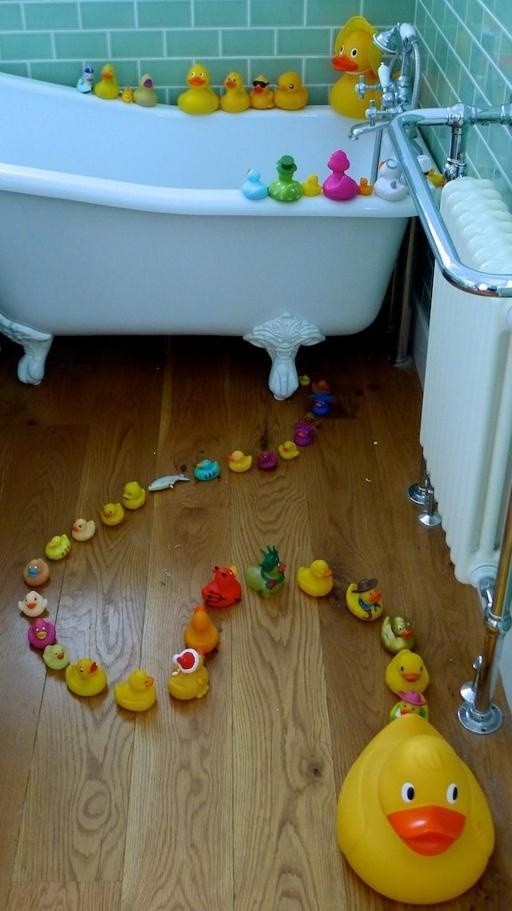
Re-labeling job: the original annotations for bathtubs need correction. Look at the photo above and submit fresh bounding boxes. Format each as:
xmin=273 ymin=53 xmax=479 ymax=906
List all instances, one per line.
xmin=0 ymin=71 xmax=447 ymax=403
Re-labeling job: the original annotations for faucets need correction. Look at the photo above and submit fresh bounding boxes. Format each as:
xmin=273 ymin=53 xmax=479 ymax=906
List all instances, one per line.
xmin=349 ymin=119 xmax=392 ymax=141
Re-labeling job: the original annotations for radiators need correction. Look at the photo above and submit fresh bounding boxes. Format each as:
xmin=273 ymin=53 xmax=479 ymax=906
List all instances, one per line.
xmin=417 ymin=173 xmax=512 ymax=733
